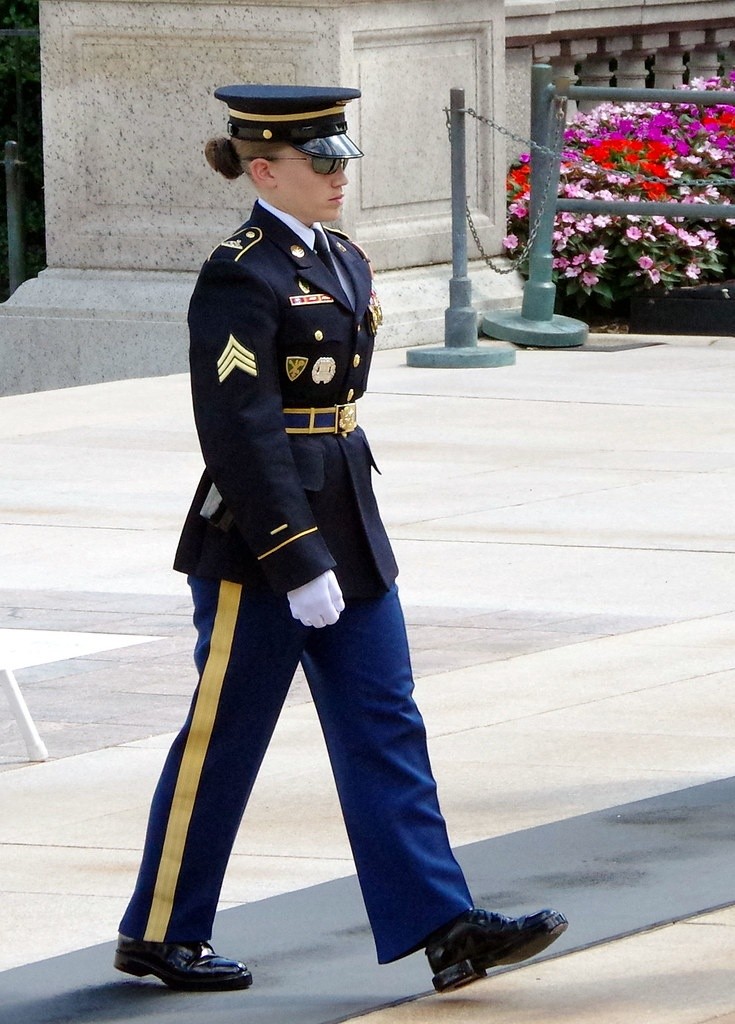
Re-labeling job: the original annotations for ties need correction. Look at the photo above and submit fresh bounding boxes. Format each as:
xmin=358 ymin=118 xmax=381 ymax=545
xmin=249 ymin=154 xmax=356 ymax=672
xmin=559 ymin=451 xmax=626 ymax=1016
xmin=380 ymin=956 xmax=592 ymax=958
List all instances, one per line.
xmin=312 ymin=228 xmax=356 ymax=317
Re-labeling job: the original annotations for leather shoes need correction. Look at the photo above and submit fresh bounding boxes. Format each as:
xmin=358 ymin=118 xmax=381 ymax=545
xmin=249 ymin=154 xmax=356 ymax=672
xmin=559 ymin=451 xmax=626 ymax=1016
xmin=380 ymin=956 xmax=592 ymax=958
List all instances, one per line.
xmin=114 ymin=931 xmax=253 ymax=992
xmin=424 ymin=908 xmax=569 ymax=993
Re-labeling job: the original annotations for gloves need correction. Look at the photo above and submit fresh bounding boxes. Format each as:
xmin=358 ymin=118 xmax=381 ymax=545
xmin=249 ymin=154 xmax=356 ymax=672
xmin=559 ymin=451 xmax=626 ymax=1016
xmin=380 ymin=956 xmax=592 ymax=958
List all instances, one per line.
xmin=287 ymin=569 xmax=345 ymax=628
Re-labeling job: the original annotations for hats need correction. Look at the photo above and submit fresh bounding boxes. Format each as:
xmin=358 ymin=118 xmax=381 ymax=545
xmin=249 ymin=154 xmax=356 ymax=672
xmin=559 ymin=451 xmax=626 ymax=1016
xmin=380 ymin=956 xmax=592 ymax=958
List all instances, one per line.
xmin=214 ymin=84 xmax=365 ymax=159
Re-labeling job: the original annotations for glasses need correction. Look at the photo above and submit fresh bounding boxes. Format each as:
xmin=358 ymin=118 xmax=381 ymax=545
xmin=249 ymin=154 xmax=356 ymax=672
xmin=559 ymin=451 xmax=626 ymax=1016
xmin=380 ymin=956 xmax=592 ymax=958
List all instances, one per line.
xmin=243 ymin=156 xmax=348 ymax=175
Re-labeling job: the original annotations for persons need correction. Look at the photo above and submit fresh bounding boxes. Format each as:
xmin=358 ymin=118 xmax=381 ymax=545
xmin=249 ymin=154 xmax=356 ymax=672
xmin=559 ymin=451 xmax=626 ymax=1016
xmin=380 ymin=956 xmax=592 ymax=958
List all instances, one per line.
xmin=113 ymin=84 xmax=570 ymax=995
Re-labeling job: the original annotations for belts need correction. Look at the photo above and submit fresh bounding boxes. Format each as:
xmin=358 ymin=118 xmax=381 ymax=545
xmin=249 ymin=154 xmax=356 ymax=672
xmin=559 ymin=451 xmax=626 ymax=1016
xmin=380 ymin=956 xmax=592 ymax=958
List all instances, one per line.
xmin=279 ymin=404 xmax=359 ymax=436
